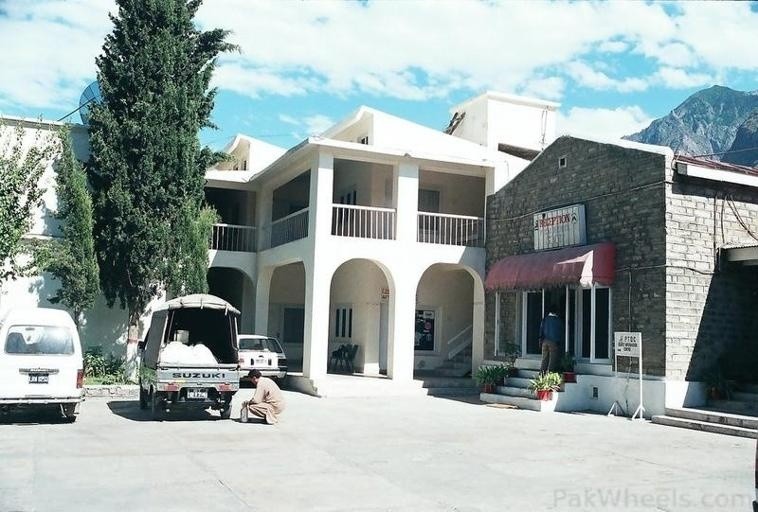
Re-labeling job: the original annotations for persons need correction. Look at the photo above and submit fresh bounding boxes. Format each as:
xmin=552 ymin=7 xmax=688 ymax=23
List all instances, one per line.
xmin=540 ymin=306 xmax=566 ymax=375
xmin=241 ymin=370 xmax=284 ymax=424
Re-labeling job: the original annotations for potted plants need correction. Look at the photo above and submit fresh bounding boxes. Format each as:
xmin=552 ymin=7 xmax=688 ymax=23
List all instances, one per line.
xmin=528 ymin=350 xmax=577 ymax=400
xmin=472 ymin=342 xmax=522 ymax=393
xmin=701 ymin=332 xmax=758 ymax=401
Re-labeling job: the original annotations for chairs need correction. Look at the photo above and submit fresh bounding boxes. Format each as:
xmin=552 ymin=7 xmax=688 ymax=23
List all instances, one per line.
xmin=330 ymin=343 xmax=359 ymax=373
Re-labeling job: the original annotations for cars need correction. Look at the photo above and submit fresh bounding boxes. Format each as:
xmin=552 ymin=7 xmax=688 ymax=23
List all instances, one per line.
xmin=236 ymin=334 xmax=288 ymax=387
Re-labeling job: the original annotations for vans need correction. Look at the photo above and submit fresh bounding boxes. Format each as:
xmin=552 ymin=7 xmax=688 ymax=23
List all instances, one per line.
xmin=0 ymin=307 xmax=87 ymax=422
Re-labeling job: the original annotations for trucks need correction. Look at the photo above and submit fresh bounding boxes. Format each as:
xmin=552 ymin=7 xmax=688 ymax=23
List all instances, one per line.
xmin=138 ymin=293 xmax=242 ymax=420
